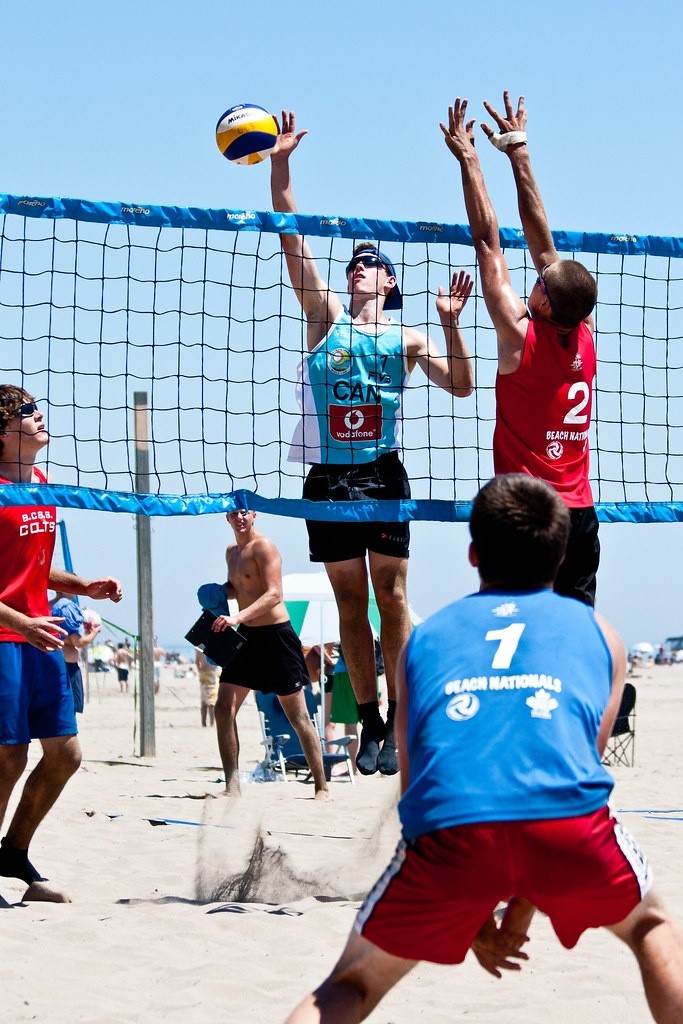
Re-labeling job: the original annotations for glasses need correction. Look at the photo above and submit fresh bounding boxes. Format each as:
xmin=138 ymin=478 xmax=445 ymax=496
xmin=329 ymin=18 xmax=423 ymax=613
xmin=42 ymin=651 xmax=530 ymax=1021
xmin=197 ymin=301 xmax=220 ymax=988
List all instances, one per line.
xmin=4 ymin=402 xmax=38 ymax=426
xmin=346 ymin=256 xmax=389 ymax=280
xmin=536 ymin=264 xmax=551 ymax=302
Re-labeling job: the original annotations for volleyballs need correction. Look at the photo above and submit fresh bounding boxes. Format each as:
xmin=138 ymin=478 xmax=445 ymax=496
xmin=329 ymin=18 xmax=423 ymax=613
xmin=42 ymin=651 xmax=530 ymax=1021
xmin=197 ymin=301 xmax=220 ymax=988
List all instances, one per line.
xmin=215 ymin=103 xmax=281 ymax=167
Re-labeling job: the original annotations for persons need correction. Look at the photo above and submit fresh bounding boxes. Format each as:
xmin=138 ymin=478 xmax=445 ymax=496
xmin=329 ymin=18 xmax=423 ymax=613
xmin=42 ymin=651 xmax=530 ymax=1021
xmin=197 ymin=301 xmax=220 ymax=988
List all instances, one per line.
xmin=284 ymin=473 xmax=683 ymax=1024
xmin=626 ymin=645 xmax=678 ymax=679
xmin=48 ymin=571 xmax=105 ymax=716
xmin=0 ymin=382 xmax=123 ymax=911
xmin=438 ymin=89 xmax=599 ymax=611
xmin=201 ymin=509 xmax=330 ymax=801
xmin=270 ymin=111 xmax=474 ymax=776
xmin=95 ymin=638 xmax=195 ymax=695
xmin=300 ymin=641 xmax=383 ymax=775
xmin=195 ymin=651 xmax=221 ymax=728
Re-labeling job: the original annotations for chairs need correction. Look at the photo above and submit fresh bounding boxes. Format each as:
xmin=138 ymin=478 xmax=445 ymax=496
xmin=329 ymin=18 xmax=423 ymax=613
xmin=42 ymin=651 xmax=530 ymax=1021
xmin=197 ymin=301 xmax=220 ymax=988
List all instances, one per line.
xmin=601 ymin=683 xmax=636 ymax=768
xmin=254 ymin=689 xmax=357 ymax=785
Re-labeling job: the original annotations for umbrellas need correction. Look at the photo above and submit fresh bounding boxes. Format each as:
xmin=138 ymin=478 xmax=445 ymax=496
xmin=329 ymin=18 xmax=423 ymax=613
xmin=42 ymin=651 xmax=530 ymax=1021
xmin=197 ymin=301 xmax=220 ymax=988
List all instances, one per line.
xmin=634 ymin=642 xmax=653 ymax=653
xmin=231 ymin=571 xmax=424 ymax=647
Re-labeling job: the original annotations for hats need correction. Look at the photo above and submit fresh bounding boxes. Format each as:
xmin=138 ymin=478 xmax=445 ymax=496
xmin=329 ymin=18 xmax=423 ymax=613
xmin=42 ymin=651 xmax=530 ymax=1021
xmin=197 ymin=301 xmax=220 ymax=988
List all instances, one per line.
xmin=352 ymin=246 xmax=403 ymax=310
